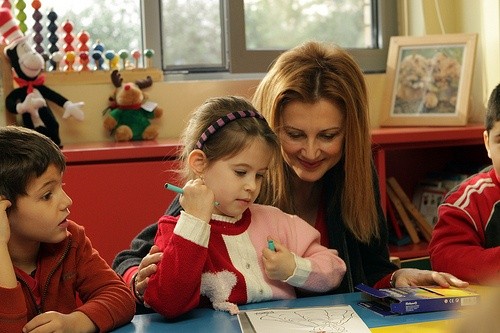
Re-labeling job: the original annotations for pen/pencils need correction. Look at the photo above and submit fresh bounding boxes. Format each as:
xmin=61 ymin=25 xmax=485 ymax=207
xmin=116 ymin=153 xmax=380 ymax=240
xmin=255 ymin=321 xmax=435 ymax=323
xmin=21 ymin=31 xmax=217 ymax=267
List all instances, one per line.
xmin=268 ymin=240 xmax=275 ymax=251
xmin=164 ymin=183 xmax=220 ymax=207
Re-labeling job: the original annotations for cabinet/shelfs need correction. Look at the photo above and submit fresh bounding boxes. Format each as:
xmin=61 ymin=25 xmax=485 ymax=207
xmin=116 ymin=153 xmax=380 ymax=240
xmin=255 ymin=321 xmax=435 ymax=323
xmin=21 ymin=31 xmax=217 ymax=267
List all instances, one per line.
xmin=61 ymin=126 xmax=489 ymax=306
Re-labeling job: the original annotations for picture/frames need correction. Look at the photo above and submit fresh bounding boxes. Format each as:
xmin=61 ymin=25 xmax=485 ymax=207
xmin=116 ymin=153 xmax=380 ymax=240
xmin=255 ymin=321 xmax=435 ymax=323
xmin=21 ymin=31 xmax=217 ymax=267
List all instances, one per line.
xmin=378 ymin=32 xmax=479 ymax=126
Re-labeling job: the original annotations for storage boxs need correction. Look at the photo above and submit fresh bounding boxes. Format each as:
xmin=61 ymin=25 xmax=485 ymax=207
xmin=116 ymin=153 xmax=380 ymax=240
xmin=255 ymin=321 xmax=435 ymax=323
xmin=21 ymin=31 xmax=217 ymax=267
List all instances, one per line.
xmin=355 ymin=286 xmax=479 ymax=318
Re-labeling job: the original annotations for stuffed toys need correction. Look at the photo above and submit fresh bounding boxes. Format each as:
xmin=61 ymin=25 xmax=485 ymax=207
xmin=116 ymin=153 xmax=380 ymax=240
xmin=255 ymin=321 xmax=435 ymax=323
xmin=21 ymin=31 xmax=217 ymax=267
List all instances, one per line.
xmin=0 ymin=10 xmax=86 ymax=148
xmin=101 ymin=68 xmax=163 ymax=142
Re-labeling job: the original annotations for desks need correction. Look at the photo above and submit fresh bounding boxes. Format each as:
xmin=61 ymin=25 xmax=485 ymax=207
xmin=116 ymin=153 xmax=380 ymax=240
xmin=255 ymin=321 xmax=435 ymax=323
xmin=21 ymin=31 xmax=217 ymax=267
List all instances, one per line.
xmin=106 ymin=286 xmax=491 ymax=333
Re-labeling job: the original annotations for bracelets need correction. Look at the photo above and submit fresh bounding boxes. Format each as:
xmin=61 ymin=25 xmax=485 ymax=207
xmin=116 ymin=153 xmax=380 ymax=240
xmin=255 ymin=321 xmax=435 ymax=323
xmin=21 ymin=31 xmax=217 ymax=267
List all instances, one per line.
xmin=389 ymin=270 xmax=395 ymax=288
xmin=130 ymin=272 xmax=145 ymax=304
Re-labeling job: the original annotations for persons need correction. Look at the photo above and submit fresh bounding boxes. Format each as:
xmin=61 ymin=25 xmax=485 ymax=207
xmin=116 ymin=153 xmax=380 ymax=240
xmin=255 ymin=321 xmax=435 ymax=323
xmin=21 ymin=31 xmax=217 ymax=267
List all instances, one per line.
xmin=136 ymin=97 xmax=346 ymax=320
xmin=0 ymin=126 xmax=136 ymax=333
xmin=113 ymin=41 xmax=468 ymax=317
xmin=428 ymin=83 xmax=500 ymax=291
